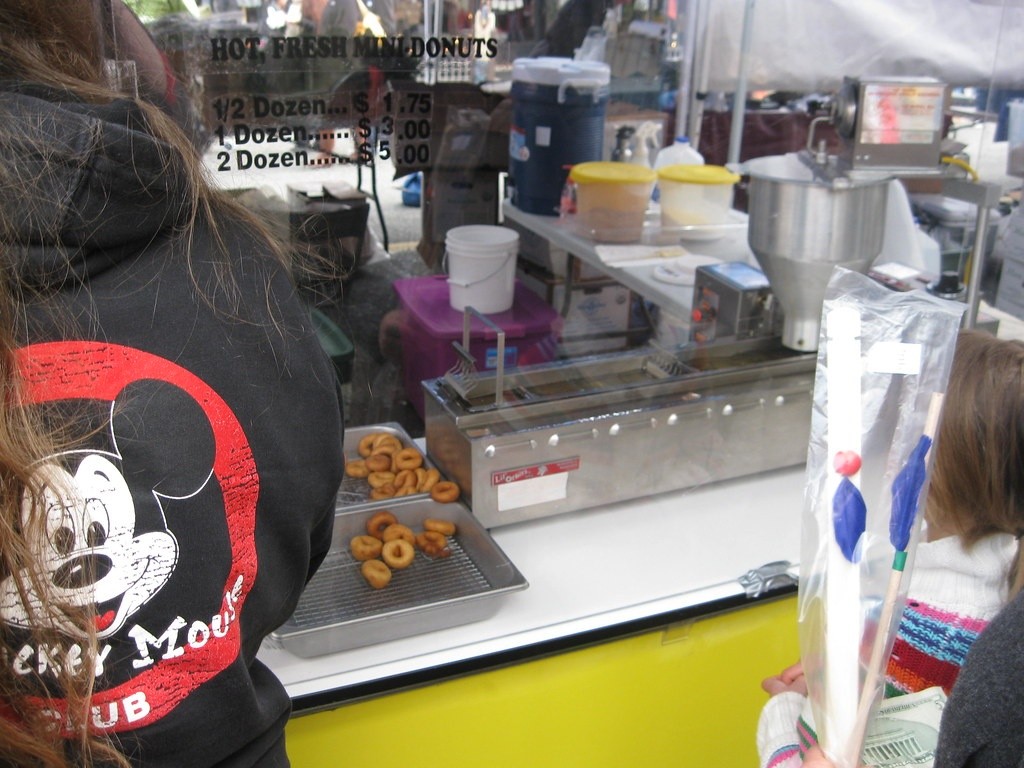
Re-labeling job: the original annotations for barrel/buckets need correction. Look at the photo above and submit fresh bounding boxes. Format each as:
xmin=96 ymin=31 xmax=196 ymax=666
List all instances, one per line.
xmin=571 ymin=162 xmax=657 ymax=241
xmin=508 ymin=56 xmax=611 ymax=215
xmin=657 ymin=165 xmax=737 ymax=243
xmin=443 ymin=224 xmax=520 ymax=314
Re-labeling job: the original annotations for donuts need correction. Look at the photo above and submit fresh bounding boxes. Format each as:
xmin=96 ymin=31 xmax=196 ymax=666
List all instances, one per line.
xmin=345 ymin=434 xmax=459 ymax=589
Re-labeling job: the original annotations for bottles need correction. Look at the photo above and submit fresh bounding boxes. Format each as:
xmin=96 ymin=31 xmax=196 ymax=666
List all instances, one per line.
xmin=655 ymin=136 xmax=704 ymax=202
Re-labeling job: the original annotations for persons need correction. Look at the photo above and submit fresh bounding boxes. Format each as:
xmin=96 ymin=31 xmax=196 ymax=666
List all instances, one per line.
xmin=754 ymin=327 xmax=1024 ymax=767
xmin=0 ymin=1 xmax=346 ymax=768
xmin=260 ymin=0 xmax=614 ymax=170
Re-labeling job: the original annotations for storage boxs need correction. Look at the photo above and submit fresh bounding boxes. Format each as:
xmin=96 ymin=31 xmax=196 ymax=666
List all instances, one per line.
xmin=392 ymin=276 xmax=585 ymax=422
xmin=387 ymin=79 xmax=511 ymax=181
xmin=602 ymin=98 xmax=669 ymax=161
xmin=422 ymin=168 xmax=498 ymax=244
xmin=517 ymin=264 xmax=632 ymax=337
xmin=503 ymin=215 xmax=609 ymax=278
